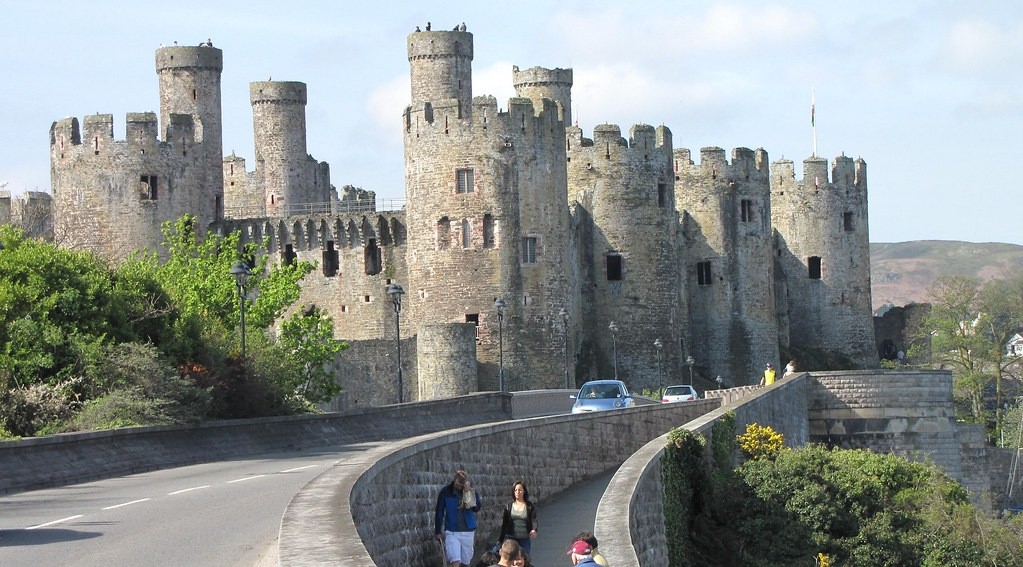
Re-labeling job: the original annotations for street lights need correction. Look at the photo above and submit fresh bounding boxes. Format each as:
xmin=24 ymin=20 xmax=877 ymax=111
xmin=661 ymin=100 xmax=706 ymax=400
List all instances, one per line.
xmin=559 ymin=307 xmax=570 ymax=388
xmin=609 ymin=321 xmax=619 ymax=380
xmin=687 ymin=356 xmax=695 ymax=386
xmin=387 ymin=284 xmax=406 ymax=403
xmin=654 ymin=338 xmax=663 ymax=401
xmin=494 ymin=298 xmax=510 ymax=392
xmin=230 ymin=262 xmax=253 ymax=359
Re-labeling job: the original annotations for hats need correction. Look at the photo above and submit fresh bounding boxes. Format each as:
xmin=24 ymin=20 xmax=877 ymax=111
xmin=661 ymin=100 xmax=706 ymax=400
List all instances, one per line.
xmin=566 ymin=540 xmax=591 ymax=555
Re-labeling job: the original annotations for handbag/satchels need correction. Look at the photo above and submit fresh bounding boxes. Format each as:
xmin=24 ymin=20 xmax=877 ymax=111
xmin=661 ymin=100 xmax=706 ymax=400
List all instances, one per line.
xmin=462 ymin=488 xmax=477 ymax=509
xmin=490 ymin=544 xmax=502 ymax=553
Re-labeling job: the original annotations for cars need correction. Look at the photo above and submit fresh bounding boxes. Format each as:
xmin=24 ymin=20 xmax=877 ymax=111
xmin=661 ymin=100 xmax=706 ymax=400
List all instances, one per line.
xmin=570 ymin=380 xmax=635 ymax=414
xmin=662 ymin=385 xmax=698 ymax=404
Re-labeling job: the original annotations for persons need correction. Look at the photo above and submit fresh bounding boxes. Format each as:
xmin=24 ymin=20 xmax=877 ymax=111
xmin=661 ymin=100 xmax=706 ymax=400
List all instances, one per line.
xmin=590 ymin=387 xmax=603 ymax=398
xmin=496 ymin=480 xmax=538 ymax=562
xmin=571 ymin=530 xmax=610 ymax=567
xmin=783 ymin=360 xmax=799 ymax=378
xmin=488 ymin=539 xmax=520 ymax=567
xmin=453 ymin=24 xmax=459 ymax=31
xmin=567 ymin=541 xmax=604 ymax=567
xmin=760 ymin=362 xmax=777 ymax=387
xmin=174 ymin=40 xmax=177 ymax=47
xmin=435 ymin=470 xmax=482 ymax=567
xmin=426 ymin=22 xmax=431 ymax=31
xmin=460 ymin=22 xmax=466 ymax=32
xmin=513 ymin=545 xmax=534 ymax=567
xmin=207 ymin=38 xmax=212 ymax=48
xmin=884 ymin=343 xmax=905 ymax=364
xmin=415 ymin=26 xmax=421 ymax=32
